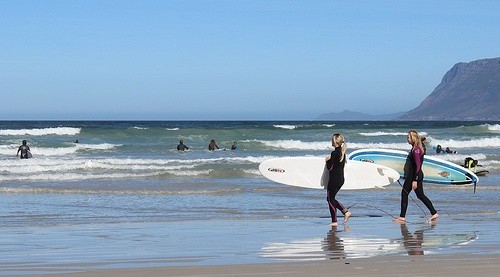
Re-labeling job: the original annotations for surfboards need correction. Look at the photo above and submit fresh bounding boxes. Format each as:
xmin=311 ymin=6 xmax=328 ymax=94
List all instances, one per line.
xmin=258 ymin=156 xmax=401 ymax=190
xmin=349 ymin=149 xmax=477 ymax=185
xmin=26 ymin=150 xmax=32 ymax=158
xmin=475 ymin=171 xmax=488 ymax=176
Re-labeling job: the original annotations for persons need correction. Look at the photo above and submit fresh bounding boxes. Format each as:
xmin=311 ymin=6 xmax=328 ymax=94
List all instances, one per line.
xmin=325 ymin=133 xmax=351 ymax=226
xmin=393 ymin=129 xmax=439 ymax=223
xmin=73 ymin=139 xmax=79 ymax=143
xmin=209 ymin=139 xmax=219 ymax=150
xmin=16 ymin=140 xmax=30 ymax=159
xmin=435 ymin=144 xmax=445 ymax=154
xmin=421 ymin=136 xmax=427 ymax=155
xmin=444 ymin=147 xmax=457 ymax=154
xmin=177 ymin=140 xmax=189 ymax=151
xmin=464 ymin=157 xmax=478 ymax=174
xmin=230 ymin=141 xmax=238 ymax=150
